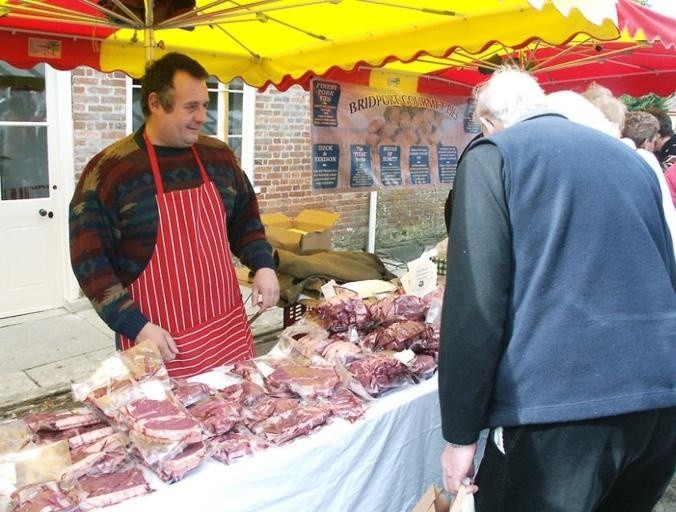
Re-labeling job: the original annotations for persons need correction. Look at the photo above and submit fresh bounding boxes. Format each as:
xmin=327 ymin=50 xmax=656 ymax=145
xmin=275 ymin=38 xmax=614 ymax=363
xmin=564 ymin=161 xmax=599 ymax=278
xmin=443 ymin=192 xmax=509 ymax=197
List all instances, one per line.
xmin=438 ymin=66 xmax=676 ymax=512
xmin=545 ymin=91 xmax=676 ymax=258
xmin=68 ymin=52 xmax=280 ymax=378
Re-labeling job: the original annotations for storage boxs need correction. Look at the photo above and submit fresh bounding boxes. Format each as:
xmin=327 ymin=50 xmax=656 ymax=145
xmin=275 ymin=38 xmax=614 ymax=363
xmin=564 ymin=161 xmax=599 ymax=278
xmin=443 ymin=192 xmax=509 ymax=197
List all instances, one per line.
xmin=260 ymin=209 xmax=340 ymax=255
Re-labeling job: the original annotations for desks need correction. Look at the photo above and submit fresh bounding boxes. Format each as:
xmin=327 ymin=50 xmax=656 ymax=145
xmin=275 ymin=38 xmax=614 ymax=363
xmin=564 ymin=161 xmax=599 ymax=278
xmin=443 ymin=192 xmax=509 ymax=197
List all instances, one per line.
xmin=0 ymin=348 xmax=488 ymax=511
xmin=236 ymin=266 xmax=325 ymax=310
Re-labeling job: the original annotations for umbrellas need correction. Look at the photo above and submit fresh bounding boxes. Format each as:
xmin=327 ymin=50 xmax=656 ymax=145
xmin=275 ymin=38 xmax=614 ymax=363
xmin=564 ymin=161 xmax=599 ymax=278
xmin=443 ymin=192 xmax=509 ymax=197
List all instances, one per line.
xmin=259 ymin=0 xmax=676 ymax=108
xmin=1 ymin=0 xmax=621 ymax=107
